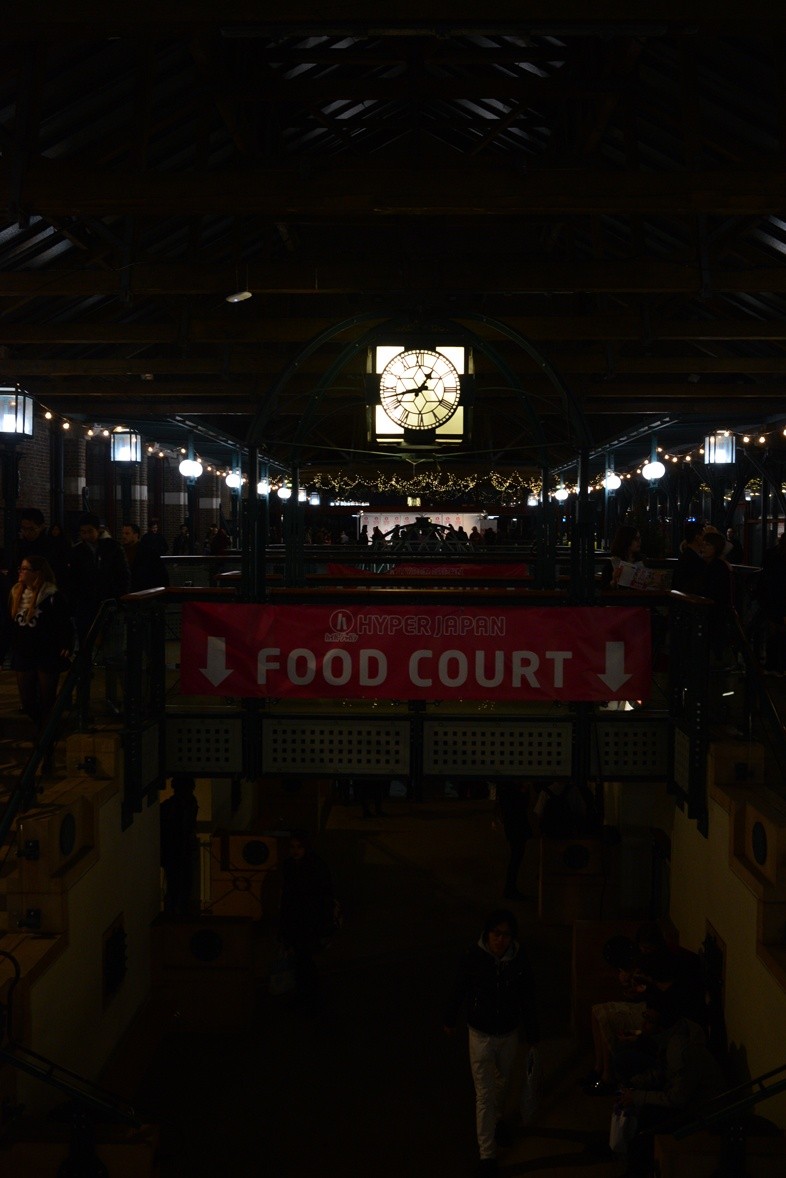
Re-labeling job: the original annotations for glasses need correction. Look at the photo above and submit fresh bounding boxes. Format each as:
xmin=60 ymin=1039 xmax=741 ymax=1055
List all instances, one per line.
xmin=488 ymin=929 xmax=513 ymax=937
xmin=17 ymin=566 xmax=36 ymax=573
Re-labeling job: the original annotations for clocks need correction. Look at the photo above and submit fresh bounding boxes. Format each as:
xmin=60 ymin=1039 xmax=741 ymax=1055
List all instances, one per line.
xmin=380 ymin=349 xmax=462 ymax=430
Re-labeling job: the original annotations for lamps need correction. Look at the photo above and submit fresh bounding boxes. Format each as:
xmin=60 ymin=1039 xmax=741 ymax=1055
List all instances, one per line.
xmin=368 ymin=319 xmax=470 ymax=452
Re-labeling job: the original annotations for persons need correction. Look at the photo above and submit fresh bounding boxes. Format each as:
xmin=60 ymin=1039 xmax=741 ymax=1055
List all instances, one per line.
xmin=1 ymin=511 xmax=786 ymax=742
xmin=493 ymin=782 xmax=532 ymax=899
xmin=444 ymin=910 xmax=547 ymax=1171
xmin=273 ymin=830 xmax=334 ymax=1017
xmin=606 ymin=999 xmax=723 ymax=1160
xmin=534 ymin=781 xmax=586 ymax=842
xmin=577 ymin=924 xmax=677 ymax=1097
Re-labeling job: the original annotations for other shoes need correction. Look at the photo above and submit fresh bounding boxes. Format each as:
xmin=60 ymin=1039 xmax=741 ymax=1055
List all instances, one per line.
xmin=495 ymin=1130 xmax=511 ymax=1149
xmin=579 ymin=1071 xmax=607 ymax=1095
xmin=473 ymin=1159 xmax=497 ymax=1177
